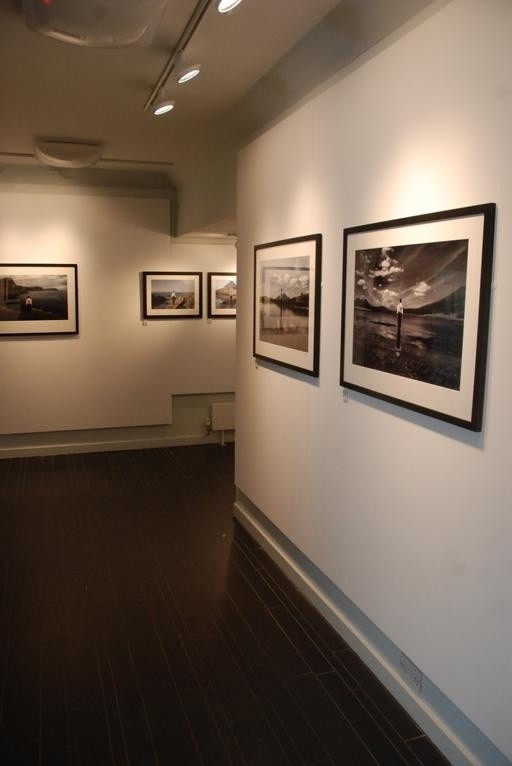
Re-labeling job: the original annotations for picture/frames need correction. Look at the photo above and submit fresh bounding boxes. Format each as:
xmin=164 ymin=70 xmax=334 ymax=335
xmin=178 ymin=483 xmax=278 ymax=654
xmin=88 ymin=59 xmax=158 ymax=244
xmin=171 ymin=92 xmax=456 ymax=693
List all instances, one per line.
xmin=253 ymin=234 xmax=322 ymax=377
xmin=339 ymin=202 xmax=495 ymax=431
xmin=142 ymin=271 xmax=202 ymax=319
xmin=0 ymin=262 xmax=79 ymax=337
xmin=207 ymin=272 xmax=237 ymax=318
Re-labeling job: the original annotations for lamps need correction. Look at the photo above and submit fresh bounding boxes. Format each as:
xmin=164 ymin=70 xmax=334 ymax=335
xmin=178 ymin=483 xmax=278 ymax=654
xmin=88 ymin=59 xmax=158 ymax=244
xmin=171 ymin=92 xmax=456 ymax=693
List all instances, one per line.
xmin=142 ymin=0 xmax=242 ymax=115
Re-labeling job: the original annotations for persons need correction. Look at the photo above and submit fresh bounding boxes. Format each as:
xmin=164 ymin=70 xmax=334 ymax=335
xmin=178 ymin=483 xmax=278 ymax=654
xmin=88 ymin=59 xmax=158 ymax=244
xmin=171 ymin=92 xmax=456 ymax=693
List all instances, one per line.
xmin=25 ymin=296 xmax=32 ymax=312
xmin=171 ymin=290 xmax=176 ymax=305
xmin=229 ymin=291 xmax=233 ymax=302
xmin=396 ymin=298 xmax=404 ymax=349
